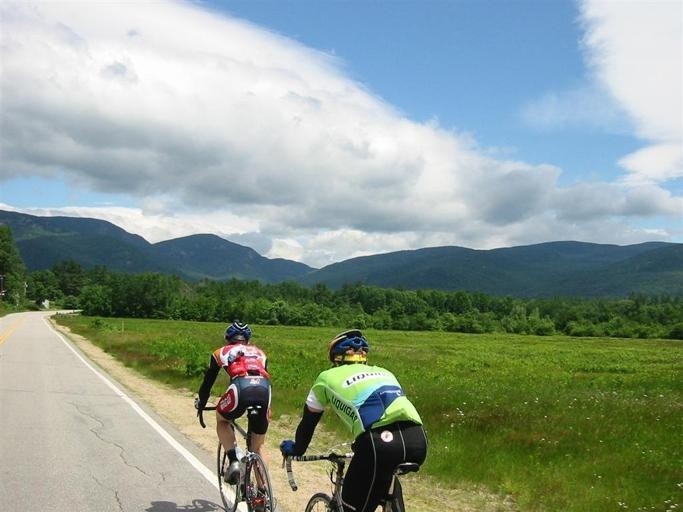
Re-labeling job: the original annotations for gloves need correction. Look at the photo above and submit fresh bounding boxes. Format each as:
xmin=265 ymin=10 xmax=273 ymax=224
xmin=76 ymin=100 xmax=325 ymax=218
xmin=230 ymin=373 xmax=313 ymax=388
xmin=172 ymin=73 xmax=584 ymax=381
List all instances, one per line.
xmin=197 ymin=398 xmax=207 ymax=410
xmin=277 ymin=440 xmax=301 ymax=459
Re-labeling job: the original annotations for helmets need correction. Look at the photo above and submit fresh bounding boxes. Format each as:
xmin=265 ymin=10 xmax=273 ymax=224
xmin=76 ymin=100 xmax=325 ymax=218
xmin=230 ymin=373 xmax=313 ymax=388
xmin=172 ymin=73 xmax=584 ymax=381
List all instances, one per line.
xmin=326 ymin=328 xmax=369 ymax=365
xmin=222 ymin=321 xmax=252 ymax=345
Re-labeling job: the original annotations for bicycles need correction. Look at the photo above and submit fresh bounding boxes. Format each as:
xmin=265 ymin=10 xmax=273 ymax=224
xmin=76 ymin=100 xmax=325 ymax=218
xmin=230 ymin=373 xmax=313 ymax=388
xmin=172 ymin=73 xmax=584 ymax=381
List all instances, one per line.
xmin=281 ymin=443 xmax=419 ymax=512
xmin=195 ymin=397 xmax=276 ymax=512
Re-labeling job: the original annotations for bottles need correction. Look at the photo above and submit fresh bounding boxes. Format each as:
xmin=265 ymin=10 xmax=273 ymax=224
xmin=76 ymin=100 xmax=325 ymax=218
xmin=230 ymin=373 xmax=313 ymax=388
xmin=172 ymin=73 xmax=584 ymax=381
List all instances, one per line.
xmin=234 ymin=445 xmax=246 ymax=461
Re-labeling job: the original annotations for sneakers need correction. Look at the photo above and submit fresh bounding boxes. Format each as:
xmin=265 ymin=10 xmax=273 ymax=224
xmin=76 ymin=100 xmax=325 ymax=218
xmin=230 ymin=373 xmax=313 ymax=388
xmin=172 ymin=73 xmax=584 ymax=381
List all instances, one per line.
xmin=222 ymin=461 xmax=241 ymax=485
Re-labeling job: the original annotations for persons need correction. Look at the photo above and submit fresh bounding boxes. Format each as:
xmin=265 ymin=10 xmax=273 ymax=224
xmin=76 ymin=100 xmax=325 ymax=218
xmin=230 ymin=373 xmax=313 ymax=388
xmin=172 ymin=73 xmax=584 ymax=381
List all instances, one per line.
xmin=189 ymin=321 xmax=272 ymax=504
xmin=279 ymin=327 xmax=427 ymax=512
